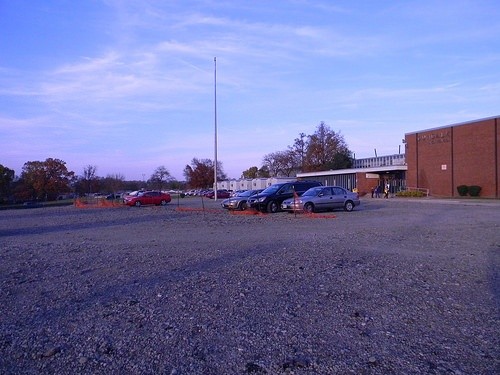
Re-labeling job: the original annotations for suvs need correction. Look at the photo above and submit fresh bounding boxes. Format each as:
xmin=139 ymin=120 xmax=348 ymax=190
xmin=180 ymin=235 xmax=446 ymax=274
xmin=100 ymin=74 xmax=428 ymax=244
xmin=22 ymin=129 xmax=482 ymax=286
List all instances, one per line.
xmin=247 ymin=180 xmax=324 ymax=213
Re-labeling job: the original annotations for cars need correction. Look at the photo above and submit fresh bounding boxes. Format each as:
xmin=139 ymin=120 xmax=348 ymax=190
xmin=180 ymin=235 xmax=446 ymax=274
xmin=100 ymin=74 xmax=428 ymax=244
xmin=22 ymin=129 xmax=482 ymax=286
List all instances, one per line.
xmin=184 ymin=189 xmax=265 ymax=211
xmin=57 ymin=193 xmax=87 ymax=200
xmin=93 ymin=190 xmax=182 ymax=207
xmin=281 ymin=186 xmax=360 ymax=213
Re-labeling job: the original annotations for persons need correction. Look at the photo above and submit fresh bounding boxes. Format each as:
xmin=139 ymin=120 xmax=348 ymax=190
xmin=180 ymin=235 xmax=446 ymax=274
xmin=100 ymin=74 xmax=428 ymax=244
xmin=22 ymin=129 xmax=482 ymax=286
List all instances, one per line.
xmin=384 ymin=187 xmax=388 ymax=199
xmin=374 ymin=186 xmax=381 ymax=198
xmin=371 ymin=186 xmax=374 ymax=198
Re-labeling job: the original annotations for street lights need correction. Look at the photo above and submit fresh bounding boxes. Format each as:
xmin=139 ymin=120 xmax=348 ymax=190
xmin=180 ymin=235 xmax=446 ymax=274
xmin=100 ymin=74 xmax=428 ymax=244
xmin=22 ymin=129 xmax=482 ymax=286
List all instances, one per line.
xmin=214 ymin=56 xmax=217 ymax=201
